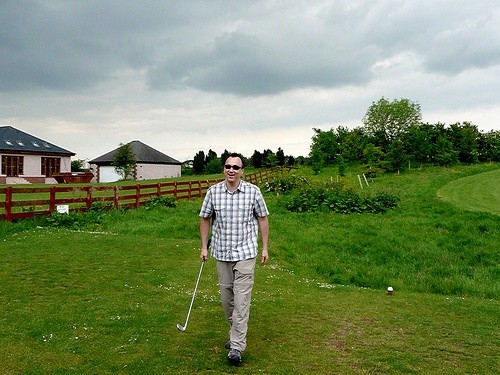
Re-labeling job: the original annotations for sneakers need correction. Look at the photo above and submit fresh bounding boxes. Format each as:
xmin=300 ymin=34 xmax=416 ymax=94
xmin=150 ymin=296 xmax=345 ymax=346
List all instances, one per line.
xmin=225 ymin=340 xmax=230 ymax=349
xmin=228 ymin=348 xmax=241 ymax=364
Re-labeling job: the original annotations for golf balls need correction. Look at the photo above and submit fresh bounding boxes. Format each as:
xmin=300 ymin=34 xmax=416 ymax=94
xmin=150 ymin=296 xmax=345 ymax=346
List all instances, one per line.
xmin=387 ymin=286 xmax=393 ymax=291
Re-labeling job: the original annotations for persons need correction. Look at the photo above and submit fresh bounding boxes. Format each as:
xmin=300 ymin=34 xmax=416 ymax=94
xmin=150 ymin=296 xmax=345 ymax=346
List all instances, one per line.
xmin=197 ymin=152 xmax=271 ymax=364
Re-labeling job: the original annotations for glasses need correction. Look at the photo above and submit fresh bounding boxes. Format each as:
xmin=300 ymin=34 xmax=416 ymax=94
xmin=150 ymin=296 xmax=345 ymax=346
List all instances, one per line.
xmin=224 ymin=165 xmax=242 ymax=170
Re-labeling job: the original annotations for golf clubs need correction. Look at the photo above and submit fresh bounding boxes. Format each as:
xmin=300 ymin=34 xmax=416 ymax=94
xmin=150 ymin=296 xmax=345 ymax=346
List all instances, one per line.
xmin=177 ymin=238 xmax=211 ymax=331
